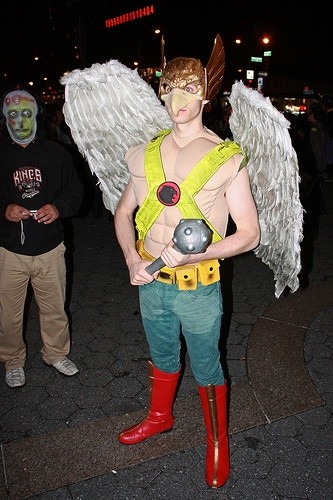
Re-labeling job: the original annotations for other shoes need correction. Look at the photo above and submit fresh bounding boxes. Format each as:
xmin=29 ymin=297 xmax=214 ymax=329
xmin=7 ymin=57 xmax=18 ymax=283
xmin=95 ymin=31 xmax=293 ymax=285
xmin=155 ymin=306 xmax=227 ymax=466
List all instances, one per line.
xmin=44 ymin=355 xmax=80 ymax=376
xmin=5 ymin=366 xmax=26 ymax=388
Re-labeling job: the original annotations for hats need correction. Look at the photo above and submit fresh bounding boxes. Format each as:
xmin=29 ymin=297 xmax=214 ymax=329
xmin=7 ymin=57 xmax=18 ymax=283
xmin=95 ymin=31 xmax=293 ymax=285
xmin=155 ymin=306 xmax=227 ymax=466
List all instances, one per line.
xmin=158 ymin=33 xmax=225 ymax=118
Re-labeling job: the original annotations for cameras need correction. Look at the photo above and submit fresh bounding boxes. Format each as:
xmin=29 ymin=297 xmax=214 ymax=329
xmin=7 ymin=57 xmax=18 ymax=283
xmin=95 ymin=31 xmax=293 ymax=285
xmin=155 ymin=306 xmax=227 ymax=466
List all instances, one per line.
xmin=22 ymin=210 xmax=39 ymax=219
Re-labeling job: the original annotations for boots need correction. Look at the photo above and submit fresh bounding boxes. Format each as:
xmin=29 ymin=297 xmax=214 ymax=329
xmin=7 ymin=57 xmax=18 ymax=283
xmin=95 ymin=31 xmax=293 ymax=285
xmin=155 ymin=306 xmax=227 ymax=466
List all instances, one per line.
xmin=197 ymin=380 xmax=231 ymax=489
xmin=118 ymin=360 xmax=184 ymax=445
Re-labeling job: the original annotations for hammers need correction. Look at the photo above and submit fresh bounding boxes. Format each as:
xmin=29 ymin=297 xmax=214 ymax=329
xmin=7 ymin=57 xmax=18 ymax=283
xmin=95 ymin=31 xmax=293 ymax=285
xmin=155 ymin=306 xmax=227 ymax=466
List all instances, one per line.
xmin=145 ymin=218 xmax=213 ymax=276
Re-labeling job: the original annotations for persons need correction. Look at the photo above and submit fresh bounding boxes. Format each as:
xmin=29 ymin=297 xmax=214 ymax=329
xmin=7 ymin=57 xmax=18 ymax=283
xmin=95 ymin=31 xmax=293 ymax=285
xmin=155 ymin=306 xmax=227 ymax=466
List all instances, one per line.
xmin=113 ymin=57 xmax=262 ymax=489
xmin=0 ymin=90 xmax=79 ymax=390
xmin=0 ymin=78 xmax=332 ymax=216
